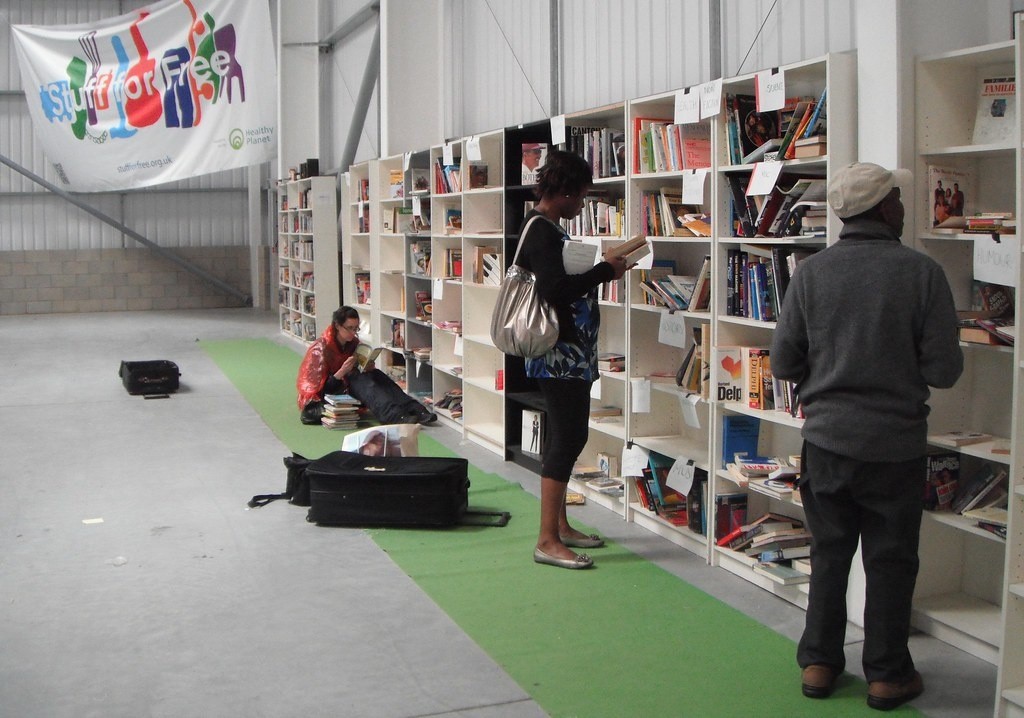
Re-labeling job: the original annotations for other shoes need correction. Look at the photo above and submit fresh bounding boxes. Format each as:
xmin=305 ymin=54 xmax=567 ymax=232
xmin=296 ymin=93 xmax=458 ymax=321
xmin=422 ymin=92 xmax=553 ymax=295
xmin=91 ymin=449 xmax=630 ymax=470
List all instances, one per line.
xmin=802 ymin=663 xmax=839 ymax=698
xmin=559 ymin=534 xmax=604 ymax=548
xmin=395 ymin=414 xmax=419 ymax=424
xmin=411 ymin=405 xmax=437 ymax=423
xmin=534 ymin=546 xmax=593 ymax=569
xmin=867 ymin=671 xmax=923 ymax=710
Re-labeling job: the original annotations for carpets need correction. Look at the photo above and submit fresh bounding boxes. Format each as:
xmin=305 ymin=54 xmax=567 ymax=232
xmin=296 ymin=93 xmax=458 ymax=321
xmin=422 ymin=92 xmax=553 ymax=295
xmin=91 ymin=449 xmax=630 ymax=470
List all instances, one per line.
xmin=195 ymin=336 xmax=928 ymax=718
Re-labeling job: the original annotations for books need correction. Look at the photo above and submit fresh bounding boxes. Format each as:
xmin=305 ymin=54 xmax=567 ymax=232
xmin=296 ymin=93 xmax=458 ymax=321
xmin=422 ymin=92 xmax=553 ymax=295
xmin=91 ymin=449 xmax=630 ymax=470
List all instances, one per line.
xmin=279 ymin=75 xmax=1018 ymax=586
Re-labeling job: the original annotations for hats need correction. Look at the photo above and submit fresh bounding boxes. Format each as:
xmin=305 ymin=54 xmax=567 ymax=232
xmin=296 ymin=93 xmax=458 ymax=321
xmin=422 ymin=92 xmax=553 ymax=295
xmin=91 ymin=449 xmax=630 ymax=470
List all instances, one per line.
xmin=829 ymin=162 xmax=912 ymax=217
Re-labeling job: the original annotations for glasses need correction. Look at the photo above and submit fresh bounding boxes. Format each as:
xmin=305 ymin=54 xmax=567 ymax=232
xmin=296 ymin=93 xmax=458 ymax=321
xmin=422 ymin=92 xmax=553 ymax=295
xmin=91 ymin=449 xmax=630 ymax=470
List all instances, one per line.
xmin=341 ymin=325 xmax=360 ymax=334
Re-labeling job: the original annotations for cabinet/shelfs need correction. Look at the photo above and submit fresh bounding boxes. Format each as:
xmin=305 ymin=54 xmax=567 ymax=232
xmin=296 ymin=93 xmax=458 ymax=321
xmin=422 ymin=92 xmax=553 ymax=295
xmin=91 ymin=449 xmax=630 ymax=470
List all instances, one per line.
xmin=913 ymin=15 xmax=1024 ymax=718
xmin=277 ymin=177 xmax=339 ymax=349
xmin=340 ymin=50 xmax=856 ymax=610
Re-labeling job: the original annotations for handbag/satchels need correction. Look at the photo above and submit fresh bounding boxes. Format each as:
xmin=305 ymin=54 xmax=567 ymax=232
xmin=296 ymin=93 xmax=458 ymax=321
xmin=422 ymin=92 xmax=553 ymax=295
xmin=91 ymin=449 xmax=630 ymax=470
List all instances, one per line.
xmin=342 ymin=423 xmax=421 ymax=458
xmin=301 ymin=400 xmax=326 ymax=424
xmin=491 ymin=215 xmax=561 ymax=358
xmin=247 ymin=452 xmax=315 ymax=508
xmin=119 ymin=360 xmax=180 ymax=399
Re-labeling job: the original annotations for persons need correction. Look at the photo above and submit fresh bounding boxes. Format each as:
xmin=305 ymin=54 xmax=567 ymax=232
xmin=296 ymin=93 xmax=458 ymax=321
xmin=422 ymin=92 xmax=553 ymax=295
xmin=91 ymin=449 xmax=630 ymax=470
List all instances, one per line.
xmin=769 ymin=161 xmax=965 ymax=708
xmin=514 ymin=151 xmax=639 ymax=569
xmin=297 ymin=306 xmax=439 ymax=426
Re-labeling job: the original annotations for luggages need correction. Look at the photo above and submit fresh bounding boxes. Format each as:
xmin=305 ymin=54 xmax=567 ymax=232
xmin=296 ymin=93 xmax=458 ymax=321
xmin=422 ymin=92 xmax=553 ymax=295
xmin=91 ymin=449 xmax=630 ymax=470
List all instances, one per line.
xmin=305 ymin=451 xmax=511 ymax=529
